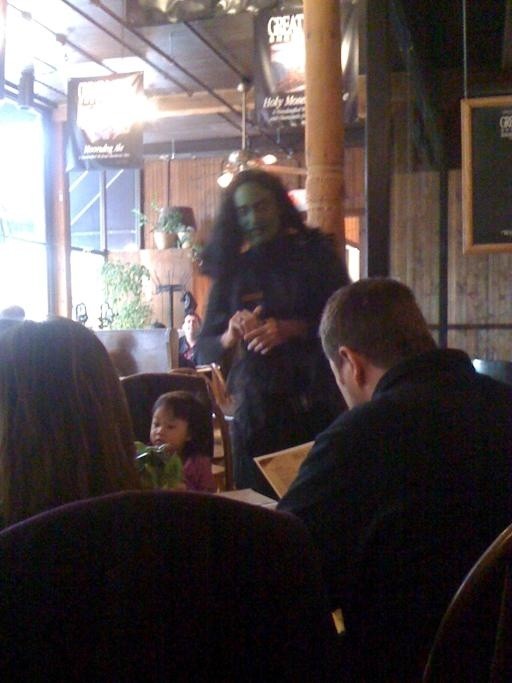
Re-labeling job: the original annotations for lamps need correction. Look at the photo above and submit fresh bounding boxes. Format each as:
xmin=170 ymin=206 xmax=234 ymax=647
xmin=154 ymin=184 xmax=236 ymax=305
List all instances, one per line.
xmin=215 ymin=82 xmax=279 ymax=189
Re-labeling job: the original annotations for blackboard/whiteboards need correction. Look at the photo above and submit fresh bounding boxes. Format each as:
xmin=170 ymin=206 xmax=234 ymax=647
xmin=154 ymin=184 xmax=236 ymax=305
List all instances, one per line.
xmin=461 ymin=95 xmax=512 ymax=256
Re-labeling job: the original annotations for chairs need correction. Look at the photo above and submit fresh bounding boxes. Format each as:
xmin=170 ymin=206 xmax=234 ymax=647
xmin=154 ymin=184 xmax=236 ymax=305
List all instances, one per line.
xmin=0 ymin=362 xmax=512 ymax=682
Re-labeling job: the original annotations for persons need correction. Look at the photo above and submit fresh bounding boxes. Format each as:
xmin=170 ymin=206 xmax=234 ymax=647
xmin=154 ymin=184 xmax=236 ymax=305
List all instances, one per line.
xmin=0 ymin=317 xmax=142 ymax=522
xmin=277 ymin=274 xmax=512 ymax=683
xmin=194 ymin=168 xmax=348 ymax=510
xmin=177 ymin=311 xmax=204 ymax=367
xmin=146 ymin=389 xmax=216 ymax=494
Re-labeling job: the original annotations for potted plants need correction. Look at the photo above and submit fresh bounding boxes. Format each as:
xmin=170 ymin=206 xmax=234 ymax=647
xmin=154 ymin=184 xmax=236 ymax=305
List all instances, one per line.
xmin=132 ymin=192 xmax=197 ymax=251
